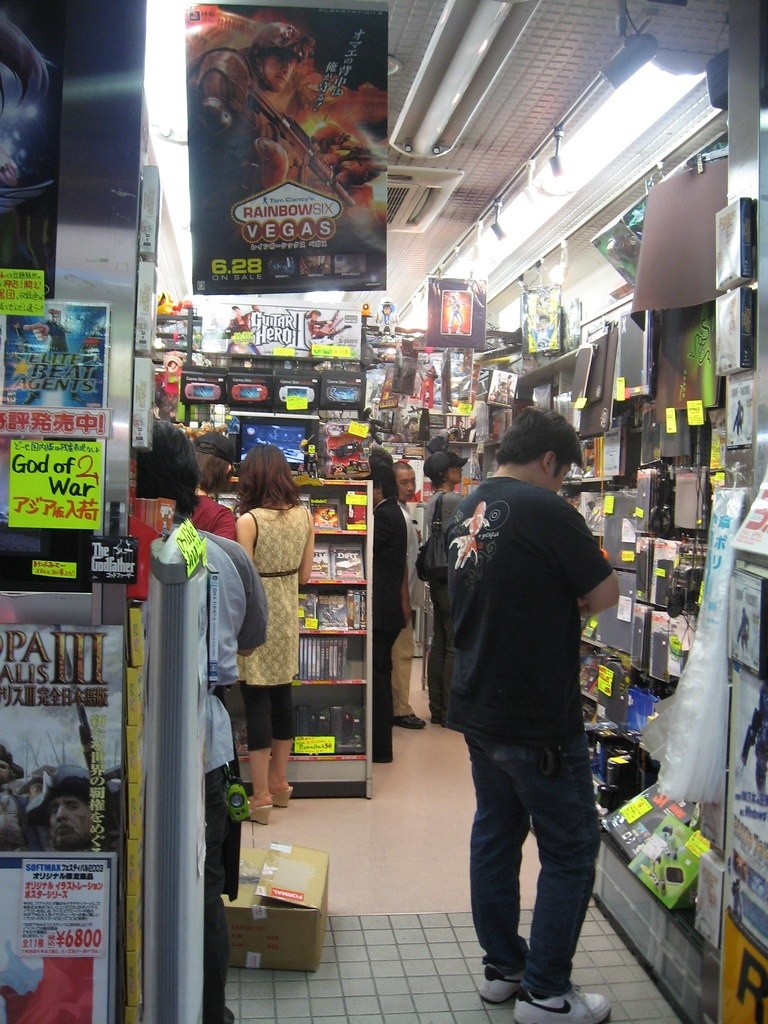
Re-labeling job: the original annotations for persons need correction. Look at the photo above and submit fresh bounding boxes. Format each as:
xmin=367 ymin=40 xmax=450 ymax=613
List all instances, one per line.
xmin=137 ymin=420 xmax=315 ymax=1024
xmin=369 ymin=447 xmax=468 ymax=764
xmin=187 ymin=22 xmax=307 ymax=191
xmin=445 ymin=405 xmax=620 ymax=1024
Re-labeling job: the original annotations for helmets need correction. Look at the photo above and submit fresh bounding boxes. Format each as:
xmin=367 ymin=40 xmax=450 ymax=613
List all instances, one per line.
xmin=252 ymin=22 xmax=304 ymax=62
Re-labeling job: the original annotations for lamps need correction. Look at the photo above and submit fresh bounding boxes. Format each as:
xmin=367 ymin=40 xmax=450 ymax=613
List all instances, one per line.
xmin=389 ymin=0 xmax=542 ymax=160
xmin=454 ymin=0 xmax=717 ymax=277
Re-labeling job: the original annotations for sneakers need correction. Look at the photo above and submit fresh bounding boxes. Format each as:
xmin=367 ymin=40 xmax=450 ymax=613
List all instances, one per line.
xmin=513 ymin=983 xmax=611 ymax=1024
xmin=478 ymin=964 xmax=522 ymax=1004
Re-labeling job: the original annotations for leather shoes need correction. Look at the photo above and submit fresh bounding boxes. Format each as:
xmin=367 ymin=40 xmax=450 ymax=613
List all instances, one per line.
xmin=394 ymin=714 xmax=426 ymax=727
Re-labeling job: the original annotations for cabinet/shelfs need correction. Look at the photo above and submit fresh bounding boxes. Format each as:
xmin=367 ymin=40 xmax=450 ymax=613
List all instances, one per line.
xmin=220 ymin=476 xmax=373 ymax=800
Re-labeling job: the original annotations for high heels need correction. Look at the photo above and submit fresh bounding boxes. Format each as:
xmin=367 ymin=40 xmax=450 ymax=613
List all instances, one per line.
xmin=245 ymin=791 xmax=271 ymax=825
xmin=271 ymin=786 xmax=293 ymax=807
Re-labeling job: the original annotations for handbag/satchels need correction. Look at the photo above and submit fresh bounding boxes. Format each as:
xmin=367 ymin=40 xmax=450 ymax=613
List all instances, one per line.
xmin=415 ymin=492 xmax=448 ymax=582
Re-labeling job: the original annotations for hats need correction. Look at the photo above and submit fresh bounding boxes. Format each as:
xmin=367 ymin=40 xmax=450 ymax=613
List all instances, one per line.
xmin=194 ymin=432 xmax=234 ymax=463
xmin=423 ymin=451 xmax=467 ymax=476
xmin=310 ymin=310 xmax=321 ymax=317
xmin=232 ymin=306 xmax=240 ymax=310
xmin=26 ymin=766 xmax=121 ymax=831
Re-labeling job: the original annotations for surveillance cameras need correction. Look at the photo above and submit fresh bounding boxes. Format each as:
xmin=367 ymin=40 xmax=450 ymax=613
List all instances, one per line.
xmin=598 ymin=32 xmax=660 ymax=90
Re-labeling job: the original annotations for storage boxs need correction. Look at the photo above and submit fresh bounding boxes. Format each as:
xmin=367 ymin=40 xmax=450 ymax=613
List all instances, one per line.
xmin=179 ymin=366 xmax=228 ymax=405
xmin=602 ymin=783 xmax=696 ymax=858
xmin=139 ymin=164 xmax=161 ymax=267
xmin=131 ymin=357 xmax=155 ymax=453
xmin=590 ymin=193 xmax=647 ymax=287
xmin=318 ymin=370 xmax=367 ymax=411
xmin=694 ymin=847 xmax=725 ymax=951
xmin=220 ymin=839 xmax=331 ymax=973
xmin=135 ymin=260 xmax=158 ymax=357
xmin=628 ymin=814 xmax=711 ymax=911
xmin=658 ymin=130 xmax=728 ymax=183
xmin=272 ymin=369 xmax=320 ymax=409
xmin=715 ymin=197 xmax=754 ymax=291
xmin=715 ymin=286 xmax=754 ymax=377
xmin=226 ymin=366 xmax=275 ymax=408
